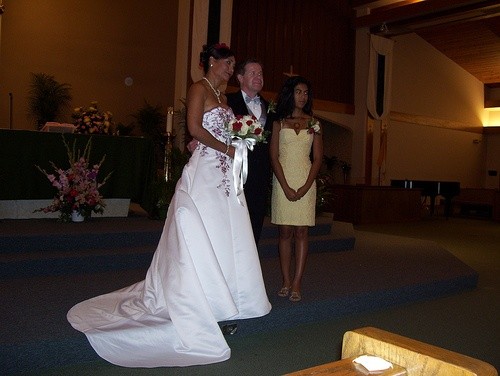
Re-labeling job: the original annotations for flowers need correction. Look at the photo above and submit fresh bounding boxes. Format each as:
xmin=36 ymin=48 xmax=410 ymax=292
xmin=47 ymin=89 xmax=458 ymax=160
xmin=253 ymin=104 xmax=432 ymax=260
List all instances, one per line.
xmin=226 ymin=113 xmax=269 ymax=147
xmin=71 ymin=99 xmax=121 ymax=136
xmin=306 ymin=115 xmax=321 ymax=135
xmin=30 ymin=127 xmax=114 ymax=222
xmin=267 ymin=100 xmax=278 ymax=114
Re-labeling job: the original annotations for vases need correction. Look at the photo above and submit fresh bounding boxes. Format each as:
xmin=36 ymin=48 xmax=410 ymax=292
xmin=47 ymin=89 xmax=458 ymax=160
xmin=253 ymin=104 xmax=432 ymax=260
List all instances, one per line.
xmin=316 ymin=173 xmax=334 ymax=219
xmin=69 ymin=208 xmax=86 ymax=222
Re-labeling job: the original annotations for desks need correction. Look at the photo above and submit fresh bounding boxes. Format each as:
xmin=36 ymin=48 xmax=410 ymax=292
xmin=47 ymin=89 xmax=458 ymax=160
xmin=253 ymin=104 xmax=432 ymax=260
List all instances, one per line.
xmin=0 ymin=126 xmax=149 ymax=219
xmin=324 ymin=183 xmax=429 ymax=226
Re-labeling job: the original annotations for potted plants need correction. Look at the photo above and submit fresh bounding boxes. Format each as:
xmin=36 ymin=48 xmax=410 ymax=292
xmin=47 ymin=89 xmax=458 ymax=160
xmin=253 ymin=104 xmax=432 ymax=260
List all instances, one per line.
xmin=131 ymin=96 xmax=192 ymax=220
xmin=25 ymin=70 xmax=74 ymax=130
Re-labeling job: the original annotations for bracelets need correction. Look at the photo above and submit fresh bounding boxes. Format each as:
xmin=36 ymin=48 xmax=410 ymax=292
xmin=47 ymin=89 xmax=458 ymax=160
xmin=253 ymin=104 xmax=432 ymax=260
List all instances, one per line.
xmin=221 ymin=144 xmax=229 ymax=158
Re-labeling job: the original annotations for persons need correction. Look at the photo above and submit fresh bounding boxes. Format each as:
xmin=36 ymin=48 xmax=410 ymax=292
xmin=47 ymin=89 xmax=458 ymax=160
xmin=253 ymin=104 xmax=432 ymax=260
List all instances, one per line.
xmin=145 ymin=42 xmax=273 ymax=321
xmin=270 ymin=75 xmax=323 ymax=301
xmin=225 ymin=57 xmax=277 ymax=244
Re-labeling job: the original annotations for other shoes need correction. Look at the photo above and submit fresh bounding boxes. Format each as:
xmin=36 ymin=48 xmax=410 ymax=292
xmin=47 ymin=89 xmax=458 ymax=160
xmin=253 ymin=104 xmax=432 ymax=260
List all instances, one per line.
xmin=289 ymin=291 xmax=304 ymax=302
xmin=278 ymin=287 xmax=290 ymax=297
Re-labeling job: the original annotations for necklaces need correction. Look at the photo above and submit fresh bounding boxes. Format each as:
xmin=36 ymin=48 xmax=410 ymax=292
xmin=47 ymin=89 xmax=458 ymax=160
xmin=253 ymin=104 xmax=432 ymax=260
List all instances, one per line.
xmin=293 ymin=115 xmax=304 ymax=129
xmin=203 ymin=76 xmax=222 ymax=103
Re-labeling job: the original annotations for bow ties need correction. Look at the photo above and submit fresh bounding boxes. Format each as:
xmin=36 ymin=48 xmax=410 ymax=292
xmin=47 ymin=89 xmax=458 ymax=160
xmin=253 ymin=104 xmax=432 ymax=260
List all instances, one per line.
xmin=245 ymin=95 xmax=260 ymax=105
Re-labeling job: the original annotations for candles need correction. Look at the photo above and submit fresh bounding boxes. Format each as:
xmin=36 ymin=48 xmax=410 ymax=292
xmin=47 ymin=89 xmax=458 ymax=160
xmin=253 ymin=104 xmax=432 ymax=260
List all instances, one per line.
xmin=437 ymin=182 xmax=440 ymax=194
